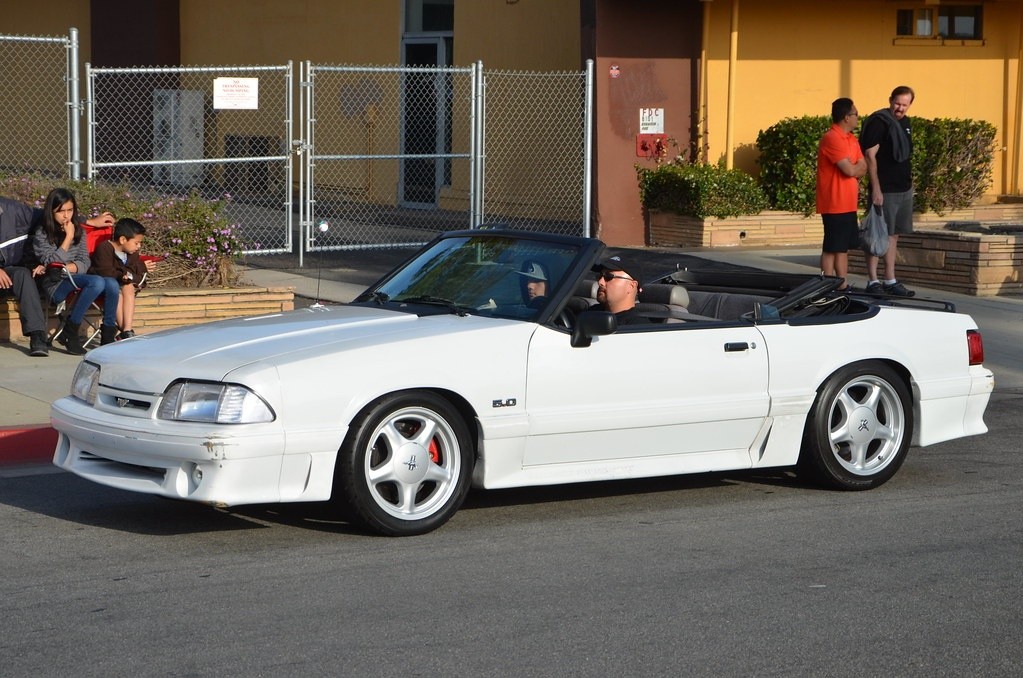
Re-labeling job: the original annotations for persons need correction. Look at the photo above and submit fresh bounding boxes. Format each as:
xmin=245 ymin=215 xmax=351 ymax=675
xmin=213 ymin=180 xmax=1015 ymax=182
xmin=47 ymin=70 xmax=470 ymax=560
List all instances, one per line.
xmin=816 ymin=98 xmax=868 ymax=292
xmin=31 ymin=188 xmax=119 ymax=355
xmin=513 ymin=253 xmax=569 ymax=308
xmin=859 ymin=86 xmax=915 ymax=297
xmin=582 ymin=253 xmax=651 ymax=324
xmin=87 ymin=218 xmax=146 ymax=341
xmin=0 ymin=196 xmax=116 ymax=357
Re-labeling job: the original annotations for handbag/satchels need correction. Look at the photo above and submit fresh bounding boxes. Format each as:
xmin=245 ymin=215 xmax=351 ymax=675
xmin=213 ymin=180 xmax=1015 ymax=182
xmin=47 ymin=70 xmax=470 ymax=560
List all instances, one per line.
xmin=859 ymin=203 xmax=890 ymax=257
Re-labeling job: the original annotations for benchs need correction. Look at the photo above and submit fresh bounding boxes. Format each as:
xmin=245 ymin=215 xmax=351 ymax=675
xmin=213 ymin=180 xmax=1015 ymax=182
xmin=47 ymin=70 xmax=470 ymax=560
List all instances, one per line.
xmin=687 ymin=291 xmax=780 ymax=322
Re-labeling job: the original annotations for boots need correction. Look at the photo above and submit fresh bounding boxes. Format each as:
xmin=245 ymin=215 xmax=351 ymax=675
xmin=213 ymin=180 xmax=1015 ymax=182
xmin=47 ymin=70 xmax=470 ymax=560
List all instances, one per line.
xmin=55 ymin=315 xmax=88 ymax=355
xmin=99 ymin=324 xmax=118 ymax=346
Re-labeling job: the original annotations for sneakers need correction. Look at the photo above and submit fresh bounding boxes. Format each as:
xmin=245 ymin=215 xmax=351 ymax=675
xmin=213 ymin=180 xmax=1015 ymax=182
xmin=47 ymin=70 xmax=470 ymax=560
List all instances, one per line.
xmin=28 ymin=330 xmax=51 ymax=357
xmin=836 ymin=284 xmax=859 ymax=296
xmin=864 ymin=279 xmax=891 ymax=300
xmin=115 ymin=329 xmax=137 ymax=341
xmin=882 ymin=280 xmax=916 ymax=297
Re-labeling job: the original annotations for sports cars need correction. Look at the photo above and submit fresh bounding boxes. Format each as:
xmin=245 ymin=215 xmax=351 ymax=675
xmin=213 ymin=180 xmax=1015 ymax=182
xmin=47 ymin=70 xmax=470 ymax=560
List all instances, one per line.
xmin=48 ymin=228 xmax=1000 ymax=541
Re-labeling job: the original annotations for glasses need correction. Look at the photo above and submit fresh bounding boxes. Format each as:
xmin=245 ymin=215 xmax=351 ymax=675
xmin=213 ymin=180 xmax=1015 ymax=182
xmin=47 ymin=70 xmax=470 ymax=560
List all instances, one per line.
xmin=848 ymin=111 xmax=858 ymax=117
xmin=595 ymin=271 xmax=634 ymax=282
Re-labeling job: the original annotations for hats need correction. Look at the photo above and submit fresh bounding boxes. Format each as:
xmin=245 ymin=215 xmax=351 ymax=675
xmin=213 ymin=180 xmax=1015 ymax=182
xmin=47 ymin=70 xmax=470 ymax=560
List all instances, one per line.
xmin=514 ymin=259 xmax=550 ymax=281
xmin=590 ymin=253 xmax=641 ymax=274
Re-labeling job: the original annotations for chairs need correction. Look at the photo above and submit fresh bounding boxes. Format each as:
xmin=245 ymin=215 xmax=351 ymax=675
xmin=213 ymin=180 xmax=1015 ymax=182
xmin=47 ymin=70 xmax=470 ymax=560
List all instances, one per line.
xmin=567 ymin=279 xmax=600 ymax=315
xmin=635 ymin=283 xmax=690 ymax=323
xmin=45 ymin=224 xmax=166 ymax=349
